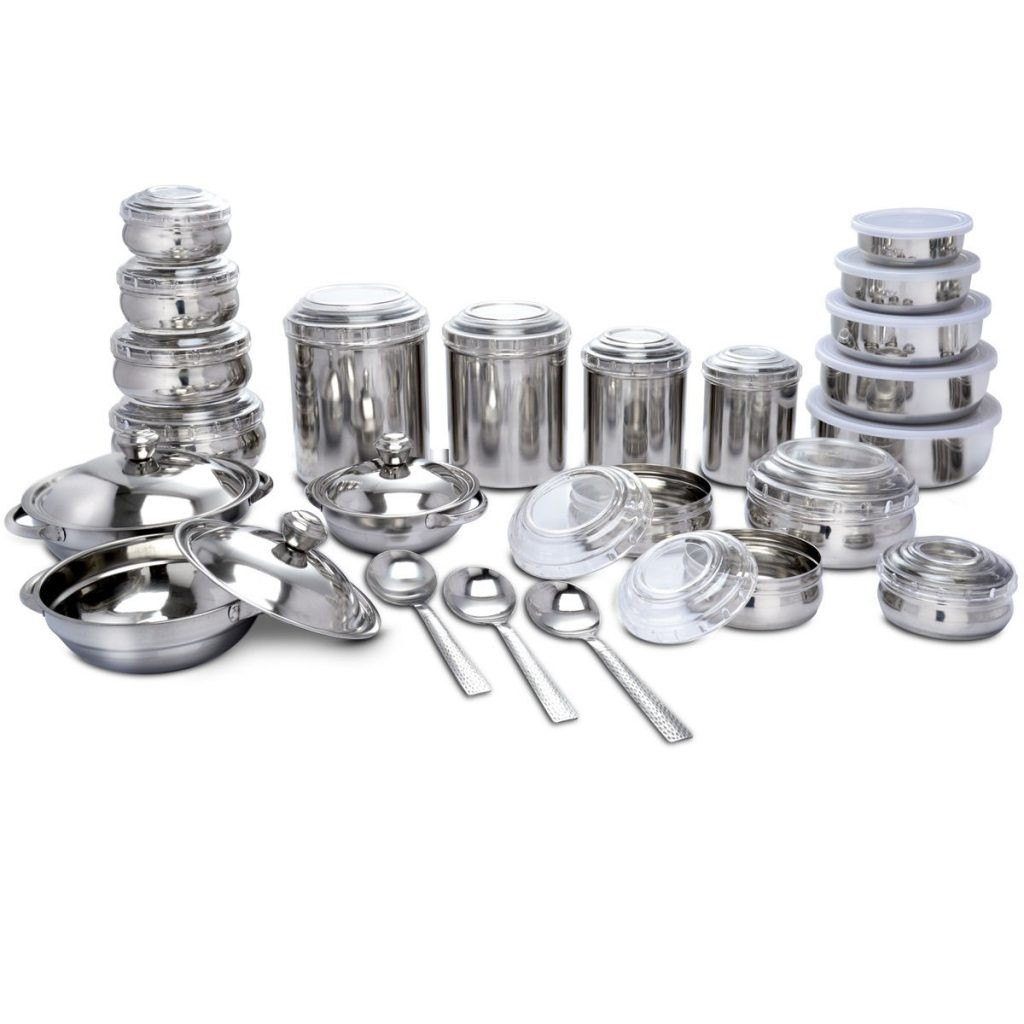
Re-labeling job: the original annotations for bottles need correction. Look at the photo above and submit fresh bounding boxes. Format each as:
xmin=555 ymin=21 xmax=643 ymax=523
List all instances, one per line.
xmin=439 ymin=301 xmax=572 ymax=489
xmin=283 ymin=283 xmax=429 ymax=484
xmin=581 ymin=328 xmax=690 ymax=470
xmin=698 ymin=346 xmax=802 ymax=487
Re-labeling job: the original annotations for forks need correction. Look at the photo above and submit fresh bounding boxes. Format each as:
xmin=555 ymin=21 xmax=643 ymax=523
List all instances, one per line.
xmin=366 ymin=548 xmax=492 ymax=696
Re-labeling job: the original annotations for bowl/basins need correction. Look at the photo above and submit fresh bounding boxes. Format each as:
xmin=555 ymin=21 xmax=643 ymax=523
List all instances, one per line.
xmin=876 ymin=535 xmax=1017 ymax=642
xmin=850 ymin=207 xmax=974 ymax=266
xmin=568 ymin=463 xmax=715 ymax=559
xmin=106 ymin=185 xmax=266 ymax=467
xmin=805 ymin=382 xmax=1001 ymax=489
xmin=816 ymin=332 xmax=998 ymax=423
xmin=833 ymin=245 xmax=981 ymax=312
xmin=681 ymin=529 xmax=822 ymax=630
xmin=823 ymin=287 xmax=992 ymax=367
xmin=744 ymin=438 xmax=918 ymax=570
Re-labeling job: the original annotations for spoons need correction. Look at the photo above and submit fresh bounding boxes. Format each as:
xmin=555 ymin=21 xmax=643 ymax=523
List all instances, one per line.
xmin=442 ymin=566 xmax=579 ymax=725
xmin=524 ymin=578 xmax=696 ymax=743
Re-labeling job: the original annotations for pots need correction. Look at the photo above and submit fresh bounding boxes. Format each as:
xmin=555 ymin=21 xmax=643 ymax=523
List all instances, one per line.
xmin=18 ymin=532 xmax=263 ymax=672
xmin=302 ymin=430 xmax=486 ymax=555
xmin=16 ymin=425 xmax=274 ymax=563
